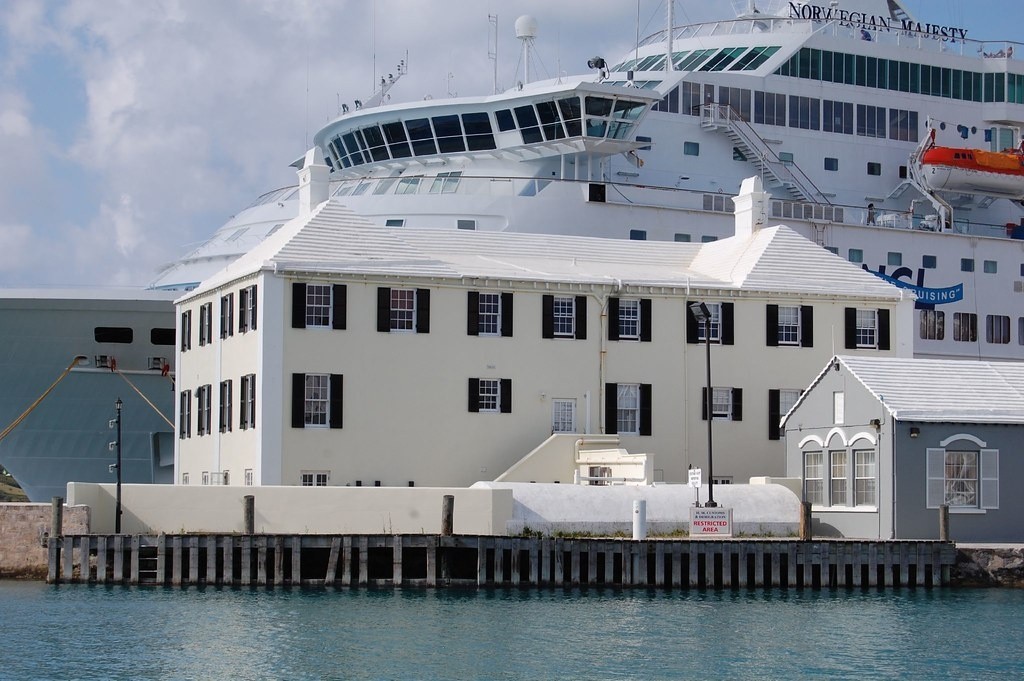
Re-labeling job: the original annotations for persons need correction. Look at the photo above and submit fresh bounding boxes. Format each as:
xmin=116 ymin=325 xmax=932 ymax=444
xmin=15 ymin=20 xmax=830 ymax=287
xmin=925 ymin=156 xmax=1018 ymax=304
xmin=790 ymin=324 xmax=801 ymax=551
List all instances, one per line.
xmin=861 ymin=29 xmax=872 ymax=41
xmin=990 ymin=47 xmax=1012 ymax=57
xmin=867 ymin=204 xmax=877 ymax=225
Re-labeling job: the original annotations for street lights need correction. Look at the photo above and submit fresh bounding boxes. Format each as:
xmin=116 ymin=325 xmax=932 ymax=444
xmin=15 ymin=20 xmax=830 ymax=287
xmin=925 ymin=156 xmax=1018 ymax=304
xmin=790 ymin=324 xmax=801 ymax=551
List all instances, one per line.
xmin=688 ymin=302 xmax=717 ymax=507
xmin=108 ymin=396 xmax=122 ymax=534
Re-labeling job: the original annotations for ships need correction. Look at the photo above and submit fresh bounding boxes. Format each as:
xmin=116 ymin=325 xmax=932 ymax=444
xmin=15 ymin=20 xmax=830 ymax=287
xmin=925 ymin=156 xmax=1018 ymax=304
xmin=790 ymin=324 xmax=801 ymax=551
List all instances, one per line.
xmin=0 ymin=0 xmax=1024 ymax=501
